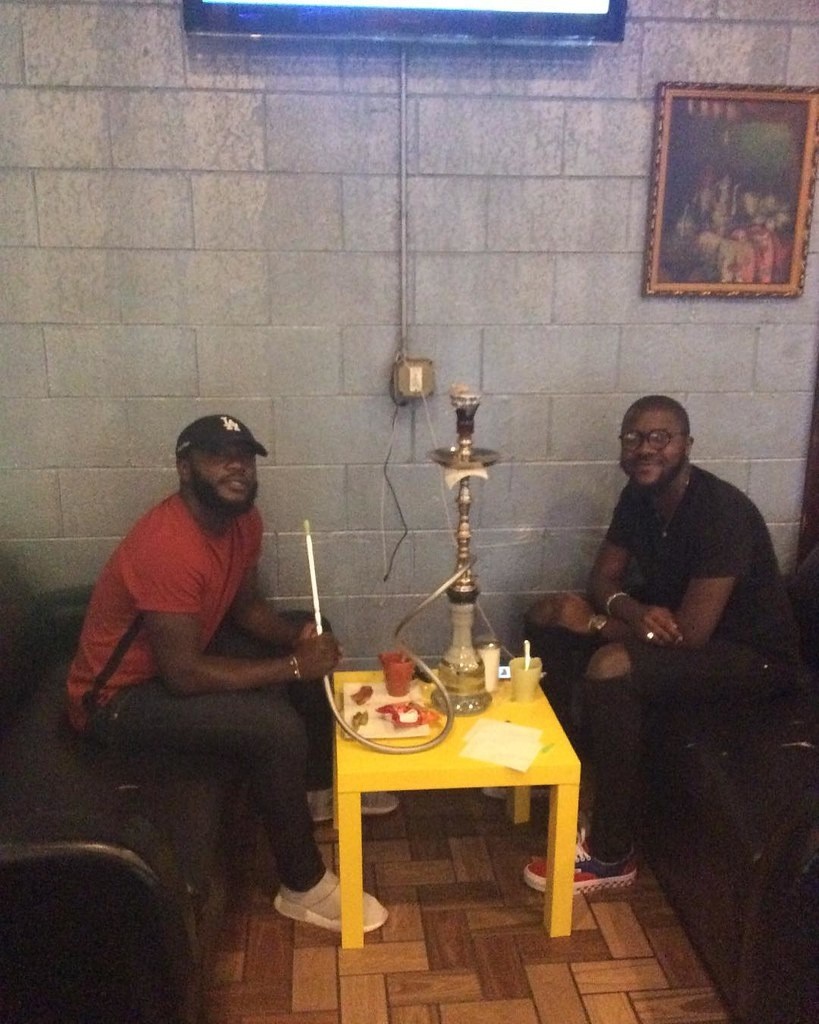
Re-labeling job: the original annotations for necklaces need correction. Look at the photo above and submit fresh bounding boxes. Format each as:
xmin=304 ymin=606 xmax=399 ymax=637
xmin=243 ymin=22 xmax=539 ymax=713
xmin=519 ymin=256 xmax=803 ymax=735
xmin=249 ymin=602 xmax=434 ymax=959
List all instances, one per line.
xmin=655 ymin=481 xmax=689 ymax=540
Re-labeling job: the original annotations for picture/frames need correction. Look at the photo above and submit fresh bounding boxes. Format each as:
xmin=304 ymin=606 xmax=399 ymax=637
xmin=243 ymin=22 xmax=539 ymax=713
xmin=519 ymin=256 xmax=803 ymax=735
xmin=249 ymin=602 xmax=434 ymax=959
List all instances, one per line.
xmin=638 ymin=80 xmax=819 ymax=299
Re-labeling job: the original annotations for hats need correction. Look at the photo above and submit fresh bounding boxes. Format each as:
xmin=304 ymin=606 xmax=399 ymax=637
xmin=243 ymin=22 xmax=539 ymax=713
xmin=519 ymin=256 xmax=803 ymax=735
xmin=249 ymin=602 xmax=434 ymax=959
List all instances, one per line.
xmin=176 ymin=415 xmax=267 ymax=461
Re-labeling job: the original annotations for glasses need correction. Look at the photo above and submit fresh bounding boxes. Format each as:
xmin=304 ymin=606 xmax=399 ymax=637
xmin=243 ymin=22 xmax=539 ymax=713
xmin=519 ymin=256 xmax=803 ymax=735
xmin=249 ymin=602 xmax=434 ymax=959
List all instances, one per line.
xmin=620 ymin=428 xmax=687 ymax=452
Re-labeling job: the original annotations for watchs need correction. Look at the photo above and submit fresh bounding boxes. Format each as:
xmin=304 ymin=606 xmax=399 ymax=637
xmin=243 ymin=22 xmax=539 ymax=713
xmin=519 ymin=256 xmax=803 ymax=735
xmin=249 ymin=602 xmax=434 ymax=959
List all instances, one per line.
xmin=589 ymin=614 xmax=608 ymax=638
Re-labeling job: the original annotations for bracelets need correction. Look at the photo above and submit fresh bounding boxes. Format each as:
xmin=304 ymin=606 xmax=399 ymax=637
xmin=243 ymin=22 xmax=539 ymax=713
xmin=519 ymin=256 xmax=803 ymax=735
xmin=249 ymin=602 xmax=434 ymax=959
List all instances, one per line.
xmin=300 ymin=622 xmax=314 ymax=638
xmin=606 ymin=592 xmax=628 ymax=615
xmin=282 ymin=655 xmax=301 ymax=684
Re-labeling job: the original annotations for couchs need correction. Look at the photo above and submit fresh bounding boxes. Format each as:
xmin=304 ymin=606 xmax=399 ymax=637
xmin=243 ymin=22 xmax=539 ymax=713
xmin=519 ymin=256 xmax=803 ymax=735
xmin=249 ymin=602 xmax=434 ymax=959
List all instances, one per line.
xmin=0 ymin=544 xmax=240 ymax=1024
xmin=578 ymin=538 xmax=819 ymax=1024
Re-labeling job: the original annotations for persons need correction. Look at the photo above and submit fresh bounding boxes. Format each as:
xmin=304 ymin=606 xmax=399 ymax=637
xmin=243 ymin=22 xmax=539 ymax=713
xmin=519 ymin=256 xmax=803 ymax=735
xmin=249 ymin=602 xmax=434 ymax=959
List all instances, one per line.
xmin=68 ymin=415 xmax=402 ymax=931
xmin=485 ymin=395 xmax=797 ymax=897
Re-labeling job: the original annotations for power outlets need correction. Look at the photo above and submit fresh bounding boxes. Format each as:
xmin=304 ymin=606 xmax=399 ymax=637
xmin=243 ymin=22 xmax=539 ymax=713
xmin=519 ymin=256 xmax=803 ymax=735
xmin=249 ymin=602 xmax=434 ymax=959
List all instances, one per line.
xmin=393 ymin=358 xmax=434 ymax=399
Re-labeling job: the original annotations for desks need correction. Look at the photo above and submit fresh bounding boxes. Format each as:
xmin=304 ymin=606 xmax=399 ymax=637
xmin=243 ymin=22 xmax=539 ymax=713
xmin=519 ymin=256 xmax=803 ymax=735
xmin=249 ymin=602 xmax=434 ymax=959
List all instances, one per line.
xmin=335 ymin=669 xmax=582 ymax=948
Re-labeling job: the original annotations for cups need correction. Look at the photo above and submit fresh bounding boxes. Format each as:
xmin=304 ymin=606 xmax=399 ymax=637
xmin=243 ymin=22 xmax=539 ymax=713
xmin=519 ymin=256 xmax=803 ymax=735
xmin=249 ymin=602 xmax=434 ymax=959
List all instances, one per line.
xmin=509 ymin=657 xmax=543 ymax=702
xmin=378 ymin=652 xmax=414 ymax=696
xmin=472 ymin=636 xmax=500 ymax=692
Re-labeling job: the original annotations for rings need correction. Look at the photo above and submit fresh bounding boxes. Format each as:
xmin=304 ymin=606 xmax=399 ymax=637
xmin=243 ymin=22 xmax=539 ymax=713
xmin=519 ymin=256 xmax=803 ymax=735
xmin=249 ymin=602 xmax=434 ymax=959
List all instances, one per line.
xmin=647 ymin=632 xmax=654 ymax=639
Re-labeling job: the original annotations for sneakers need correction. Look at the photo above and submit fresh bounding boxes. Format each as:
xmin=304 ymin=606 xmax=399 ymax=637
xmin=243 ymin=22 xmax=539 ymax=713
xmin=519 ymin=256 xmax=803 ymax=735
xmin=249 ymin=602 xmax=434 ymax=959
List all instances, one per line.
xmin=483 ymin=783 xmax=549 ymax=800
xmin=308 ymin=784 xmax=400 ymax=821
xmin=274 ymin=867 xmax=389 ymax=936
xmin=521 ymin=828 xmax=642 ymax=894
